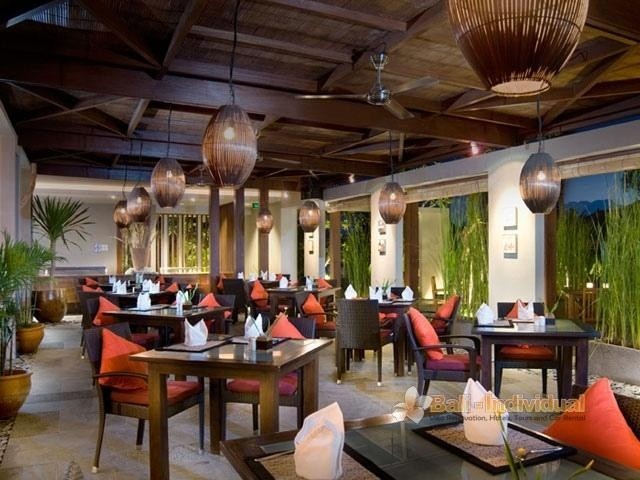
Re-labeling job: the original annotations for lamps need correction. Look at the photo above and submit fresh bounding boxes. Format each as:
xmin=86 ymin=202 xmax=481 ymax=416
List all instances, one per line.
xmin=378 ymin=130 xmax=407 ymax=225
xmin=299 ymin=175 xmax=321 ymax=232
xmin=112 ymin=132 xmax=133 ymax=228
xmin=256 ymin=177 xmax=274 ymax=234
xmin=519 ymin=97 xmax=562 ymax=214
xmin=447 ymin=0 xmax=590 ymax=97
xmin=126 ymin=122 xmax=152 ymax=223
xmin=150 ymin=103 xmax=186 ymax=209
xmin=202 ymin=0 xmax=259 ymax=189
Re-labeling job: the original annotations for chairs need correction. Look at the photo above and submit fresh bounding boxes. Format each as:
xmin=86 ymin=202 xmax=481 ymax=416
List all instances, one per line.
xmin=568 ymin=384 xmax=640 ymax=441
xmin=331 ymin=286 xmax=601 ymax=415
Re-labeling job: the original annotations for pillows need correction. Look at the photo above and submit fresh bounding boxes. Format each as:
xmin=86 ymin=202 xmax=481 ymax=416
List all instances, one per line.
xmin=542 ymin=377 xmax=640 ymax=472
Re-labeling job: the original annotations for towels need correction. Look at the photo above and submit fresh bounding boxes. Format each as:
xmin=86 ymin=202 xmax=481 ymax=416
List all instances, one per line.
xmin=460 ymin=377 xmax=509 ymax=446
xmin=293 ymin=401 xmax=346 ymax=480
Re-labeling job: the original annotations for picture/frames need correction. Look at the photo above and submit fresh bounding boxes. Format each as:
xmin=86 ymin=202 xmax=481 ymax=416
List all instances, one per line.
xmin=502 ymin=233 xmax=517 ymax=255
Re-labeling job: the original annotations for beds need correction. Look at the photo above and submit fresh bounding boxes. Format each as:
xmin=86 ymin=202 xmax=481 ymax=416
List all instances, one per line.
xmin=217 ymin=402 xmax=640 ymax=480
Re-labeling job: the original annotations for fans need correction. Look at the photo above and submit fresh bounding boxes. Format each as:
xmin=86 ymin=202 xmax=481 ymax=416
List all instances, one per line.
xmin=295 ymin=41 xmax=440 ymax=120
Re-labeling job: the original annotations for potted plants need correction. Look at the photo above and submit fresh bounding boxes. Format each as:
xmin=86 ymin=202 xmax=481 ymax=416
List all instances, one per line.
xmin=16 ymin=239 xmax=45 ymax=355
xmin=110 ymin=222 xmax=158 ymax=274
xmin=0 ymin=229 xmax=33 ymax=418
xmin=32 ymin=194 xmax=96 ymax=323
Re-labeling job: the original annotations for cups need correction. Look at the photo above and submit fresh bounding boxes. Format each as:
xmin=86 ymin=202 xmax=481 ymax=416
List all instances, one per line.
xmin=535 ymin=316 xmax=545 ymax=326
xmin=249 ymin=337 xmax=256 ymax=351
xmin=176 ymin=303 xmax=183 ymax=315
